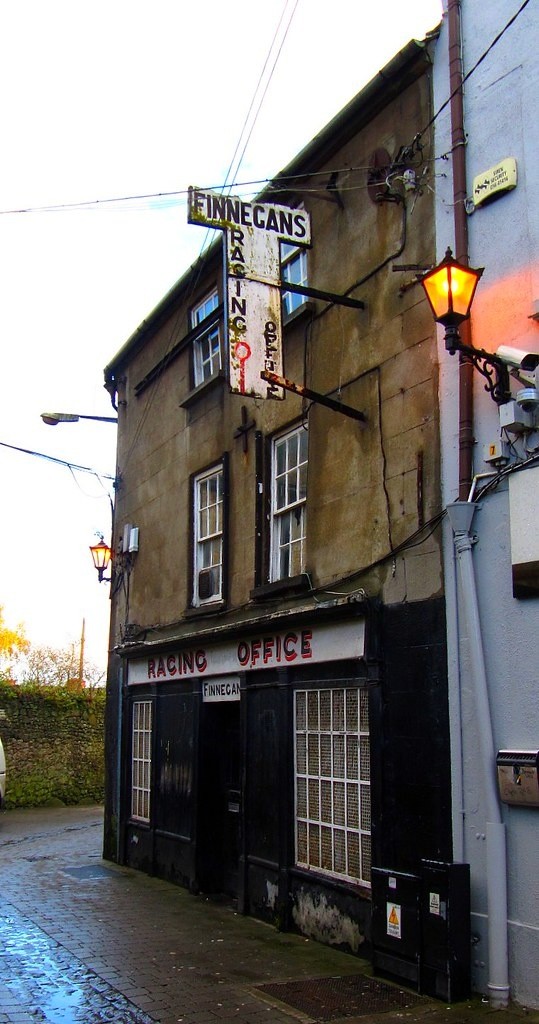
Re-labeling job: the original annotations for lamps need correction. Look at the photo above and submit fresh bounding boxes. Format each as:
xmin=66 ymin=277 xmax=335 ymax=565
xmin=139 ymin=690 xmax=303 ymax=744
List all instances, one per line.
xmin=89 ymin=531 xmax=116 ymax=583
xmin=40 ymin=412 xmax=118 ymax=426
xmin=416 ymin=245 xmax=516 ymax=414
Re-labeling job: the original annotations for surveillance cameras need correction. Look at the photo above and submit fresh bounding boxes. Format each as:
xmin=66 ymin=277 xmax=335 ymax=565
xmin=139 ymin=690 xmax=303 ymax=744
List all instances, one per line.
xmin=498 ymin=346 xmax=539 ymax=371
